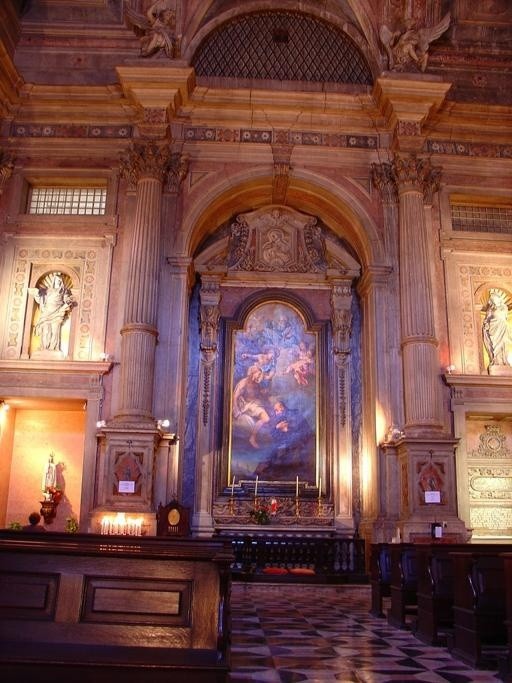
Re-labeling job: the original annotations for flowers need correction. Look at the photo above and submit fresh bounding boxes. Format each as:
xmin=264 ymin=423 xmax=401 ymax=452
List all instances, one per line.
xmin=252 ymin=503 xmax=271 ymax=525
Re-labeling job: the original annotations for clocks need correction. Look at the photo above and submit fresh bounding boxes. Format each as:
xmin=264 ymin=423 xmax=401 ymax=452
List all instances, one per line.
xmin=155 ymin=498 xmax=192 ymax=536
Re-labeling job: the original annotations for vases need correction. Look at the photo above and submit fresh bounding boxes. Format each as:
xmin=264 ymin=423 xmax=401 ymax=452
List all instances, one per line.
xmin=256 ymin=511 xmax=267 ymax=525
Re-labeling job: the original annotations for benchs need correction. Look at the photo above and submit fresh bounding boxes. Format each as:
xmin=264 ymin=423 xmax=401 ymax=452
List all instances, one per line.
xmin=365 ymin=542 xmax=510 ymax=681
xmin=0 ymin=529 xmax=237 ymax=682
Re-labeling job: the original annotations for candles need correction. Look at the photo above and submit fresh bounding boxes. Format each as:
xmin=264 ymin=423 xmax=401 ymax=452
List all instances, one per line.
xmin=231 ymin=474 xmax=322 ymax=498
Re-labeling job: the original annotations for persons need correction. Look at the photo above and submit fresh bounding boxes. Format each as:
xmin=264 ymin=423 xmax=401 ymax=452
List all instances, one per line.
xmin=476 ymin=287 xmax=511 ymax=367
xmin=25 ymin=270 xmax=75 ymax=351
xmin=235 ymin=314 xmax=315 ymax=474
